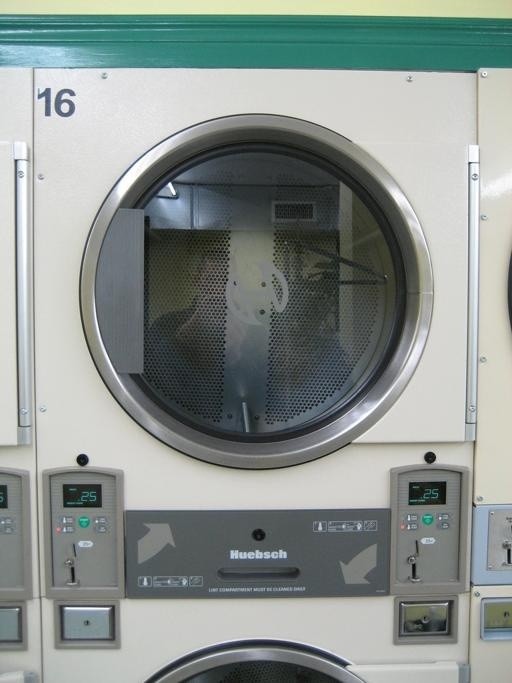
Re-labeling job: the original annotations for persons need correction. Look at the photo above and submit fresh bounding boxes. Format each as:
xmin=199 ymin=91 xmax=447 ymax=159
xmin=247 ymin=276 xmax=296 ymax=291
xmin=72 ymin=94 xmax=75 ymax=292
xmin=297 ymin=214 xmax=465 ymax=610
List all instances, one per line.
xmin=145 ymin=251 xmax=228 ymax=423
